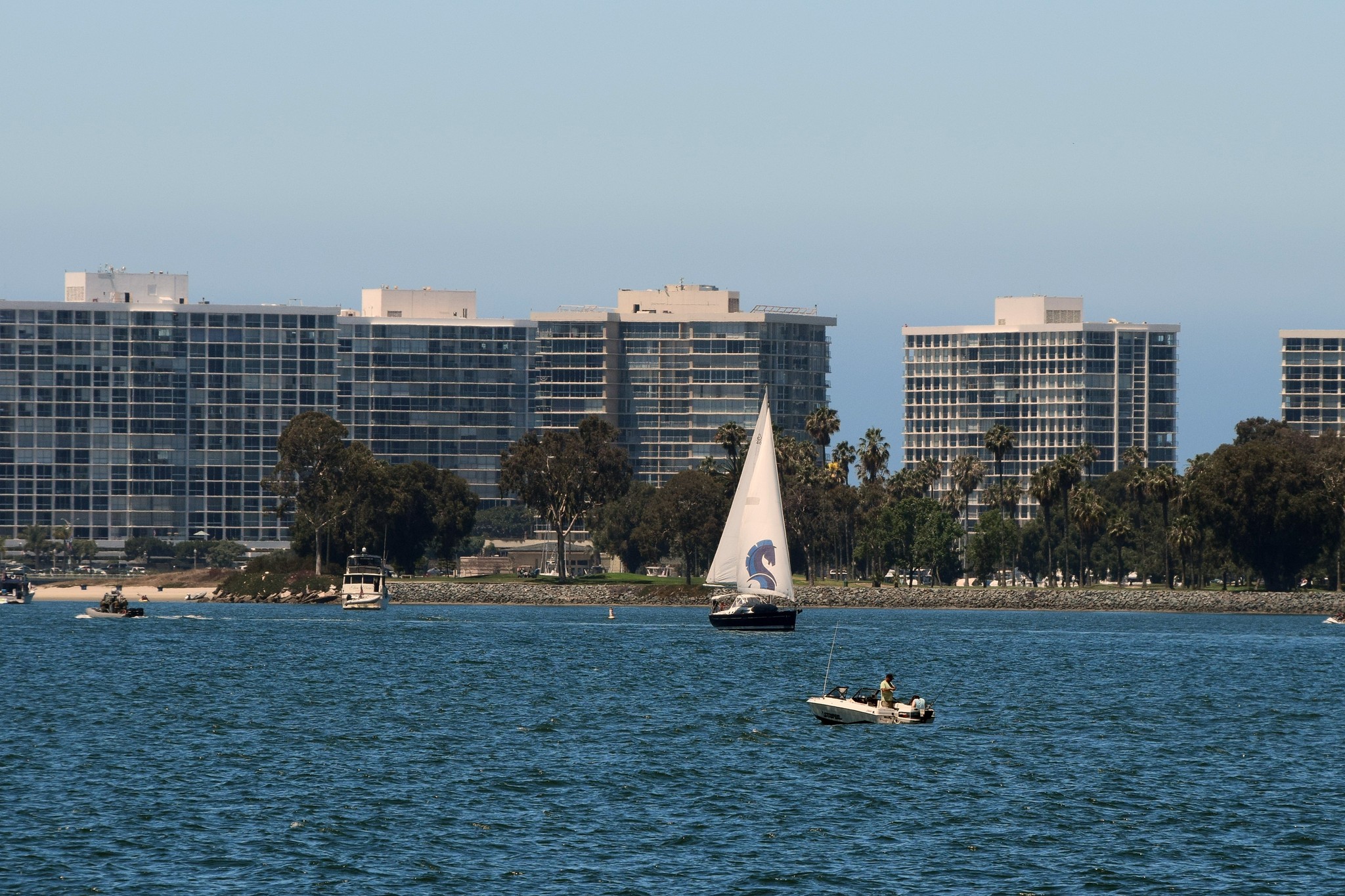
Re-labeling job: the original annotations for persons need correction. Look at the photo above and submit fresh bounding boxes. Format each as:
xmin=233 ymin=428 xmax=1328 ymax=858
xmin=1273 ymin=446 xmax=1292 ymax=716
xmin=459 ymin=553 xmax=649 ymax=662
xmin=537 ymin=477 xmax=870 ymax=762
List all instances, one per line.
xmin=100 ymin=598 xmax=108 ymax=611
xmin=141 ymin=594 xmax=147 ymax=600
xmin=711 ymin=599 xmax=719 ymax=613
xmin=719 ymin=601 xmax=726 ymax=611
xmin=880 ymin=674 xmax=896 ymax=708
xmin=112 ymin=599 xmax=120 ymax=613
xmin=120 ymin=598 xmax=128 ymax=610
xmin=911 ymin=695 xmax=926 ymax=709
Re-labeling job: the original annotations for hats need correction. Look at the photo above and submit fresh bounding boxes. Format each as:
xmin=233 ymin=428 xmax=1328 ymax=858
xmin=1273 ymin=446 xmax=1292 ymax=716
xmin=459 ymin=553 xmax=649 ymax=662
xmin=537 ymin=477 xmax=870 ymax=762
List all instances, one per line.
xmin=886 ymin=673 xmax=894 ymax=679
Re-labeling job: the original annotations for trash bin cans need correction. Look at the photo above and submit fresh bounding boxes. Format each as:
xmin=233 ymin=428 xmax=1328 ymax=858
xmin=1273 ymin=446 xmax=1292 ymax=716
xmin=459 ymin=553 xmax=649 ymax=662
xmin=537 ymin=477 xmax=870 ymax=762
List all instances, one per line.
xmin=115 ymin=585 xmax=122 ymax=591
xmin=844 ymin=580 xmax=848 ymax=586
xmin=157 ymin=586 xmax=163 ymax=592
xmin=80 ymin=584 xmax=87 ymax=590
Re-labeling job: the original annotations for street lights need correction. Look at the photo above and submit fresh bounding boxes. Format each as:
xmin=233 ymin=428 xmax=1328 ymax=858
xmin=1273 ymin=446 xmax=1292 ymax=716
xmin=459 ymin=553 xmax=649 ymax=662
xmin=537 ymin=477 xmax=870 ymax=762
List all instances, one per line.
xmin=193 ymin=549 xmax=197 ymax=575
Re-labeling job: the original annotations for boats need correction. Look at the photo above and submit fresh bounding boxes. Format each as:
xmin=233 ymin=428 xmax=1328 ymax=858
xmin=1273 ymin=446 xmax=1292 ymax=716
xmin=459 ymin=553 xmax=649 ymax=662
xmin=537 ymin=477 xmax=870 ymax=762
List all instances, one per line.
xmin=139 ymin=597 xmax=148 ymax=602
xmin=185 ymin=591 xmax=207 ymax=600
xmin=806 ymin=686 xmax=937 ymax=725
xmin=646 ymin=566 xmax=674 ymax=578
xmin=0 ymin=562 xmax=35 ymax=604
xmin=86 ymin=607 xmax=128 ymax=618
xmin=340 ymin=553 xmax=394 ymax=610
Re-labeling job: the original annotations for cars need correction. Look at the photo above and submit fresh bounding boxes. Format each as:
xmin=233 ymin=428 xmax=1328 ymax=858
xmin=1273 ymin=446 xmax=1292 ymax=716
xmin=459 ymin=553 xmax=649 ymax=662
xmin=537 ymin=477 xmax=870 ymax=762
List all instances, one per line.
xmin=74 ymin=565 xmax=95 ymax=574
xmin=427 ymin=567 xmax=453 ymax=575
xmin=129 ymin=567 xmax=146 ymax=575
xmin=517 ymin=566 xmax=535 ymax=578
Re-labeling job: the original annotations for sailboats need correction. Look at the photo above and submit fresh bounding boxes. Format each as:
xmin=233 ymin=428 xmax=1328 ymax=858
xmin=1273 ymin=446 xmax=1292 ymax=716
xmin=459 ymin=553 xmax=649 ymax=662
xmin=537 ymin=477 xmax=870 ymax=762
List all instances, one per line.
xmin=539 ymin=520 xmax=571 ymax=579
xmin=702 ymin=384 xmax=798 ymax=631
